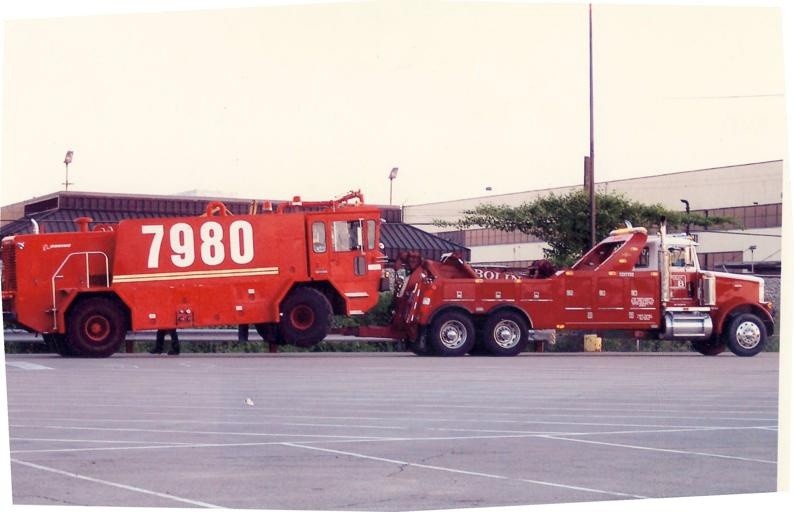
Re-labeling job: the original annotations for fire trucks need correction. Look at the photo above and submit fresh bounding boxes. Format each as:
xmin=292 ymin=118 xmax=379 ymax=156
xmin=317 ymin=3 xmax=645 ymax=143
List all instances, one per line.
xmin=2 ymin=193 xmax=387 ymax=357
xmin=394 ymin=225 xmax=775 ymax=355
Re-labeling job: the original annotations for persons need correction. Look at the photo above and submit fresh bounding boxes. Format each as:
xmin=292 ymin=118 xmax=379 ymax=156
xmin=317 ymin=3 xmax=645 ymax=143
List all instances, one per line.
xmin=147 ymin=329 xmax=180 ymax=355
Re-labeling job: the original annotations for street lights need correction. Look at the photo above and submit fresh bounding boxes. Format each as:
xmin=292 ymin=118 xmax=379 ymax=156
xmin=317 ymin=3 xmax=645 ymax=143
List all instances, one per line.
xmin=63 ymin=150 xmax=75 ymax=191
xmin=387 ymin=166 xmax=398 ymax=205
